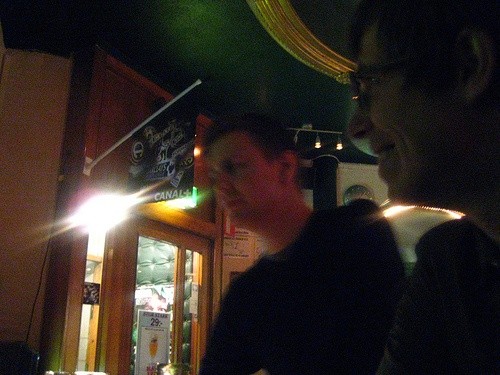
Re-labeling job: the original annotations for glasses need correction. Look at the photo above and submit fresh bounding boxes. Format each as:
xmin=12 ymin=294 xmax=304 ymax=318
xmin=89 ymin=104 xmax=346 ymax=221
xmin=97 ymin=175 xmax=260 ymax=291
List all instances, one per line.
xmin=349 ymin=59 xmax=405 ymax=109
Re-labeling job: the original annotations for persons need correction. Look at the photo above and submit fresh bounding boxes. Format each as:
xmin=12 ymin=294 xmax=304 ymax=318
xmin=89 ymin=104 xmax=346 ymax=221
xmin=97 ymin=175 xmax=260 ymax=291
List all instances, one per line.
xmin=346 ymin=1 xmax=500 ymax=375
xmin=197 ymin=111 xmax=405 ymax=375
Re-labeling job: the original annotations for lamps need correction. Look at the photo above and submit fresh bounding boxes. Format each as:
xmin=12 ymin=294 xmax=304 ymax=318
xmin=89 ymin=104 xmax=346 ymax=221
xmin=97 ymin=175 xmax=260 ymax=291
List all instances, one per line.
xmin=293 ymin=130 xmax=299 ymax=143
xmin=336 ymin=134 xmax=343 ymax=150
xmin=315 ymin=131 xmax=321 ymax=148
